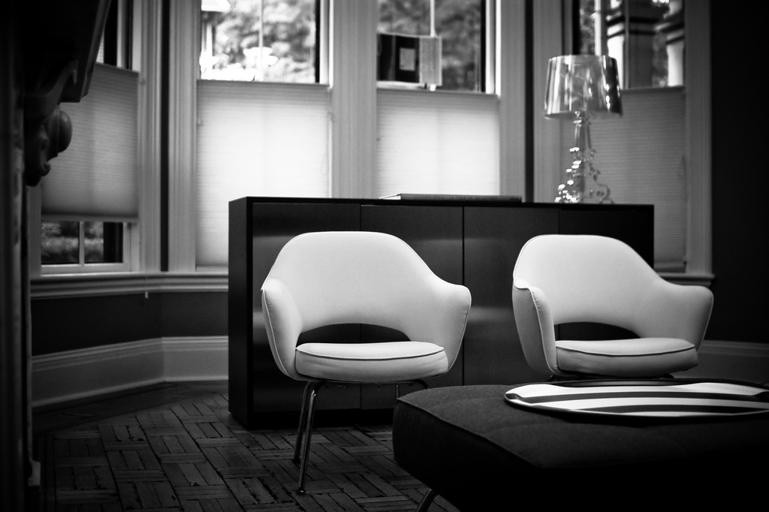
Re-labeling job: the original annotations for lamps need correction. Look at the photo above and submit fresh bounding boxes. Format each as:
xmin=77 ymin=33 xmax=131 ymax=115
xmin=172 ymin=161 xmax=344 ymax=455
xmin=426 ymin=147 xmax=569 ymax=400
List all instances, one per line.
xmin=542 ymin=54 xmax=626 ymax=204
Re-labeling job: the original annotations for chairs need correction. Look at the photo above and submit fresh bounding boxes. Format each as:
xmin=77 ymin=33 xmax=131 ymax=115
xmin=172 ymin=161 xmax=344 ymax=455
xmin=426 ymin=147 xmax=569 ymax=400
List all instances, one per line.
xmin=512 ymin=234 xmax=715 ymax=387
xmin=260 ymin=231 xmax=472 ymax=497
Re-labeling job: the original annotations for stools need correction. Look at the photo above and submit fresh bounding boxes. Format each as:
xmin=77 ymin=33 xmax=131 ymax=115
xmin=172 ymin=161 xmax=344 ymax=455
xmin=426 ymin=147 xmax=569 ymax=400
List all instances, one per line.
xmin=391 ymin=378 xmax=769 ymax=512
